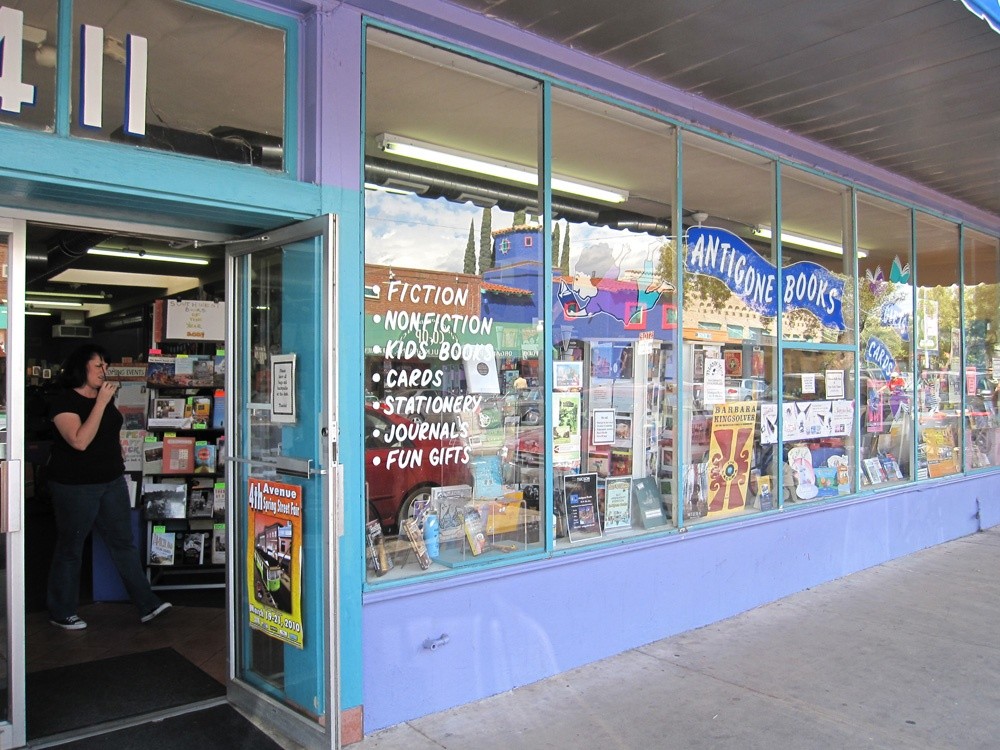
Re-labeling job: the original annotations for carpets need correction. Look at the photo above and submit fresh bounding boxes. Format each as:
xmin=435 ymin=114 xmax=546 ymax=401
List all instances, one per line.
xmin=0 ymin=644 xmax=226 ymax=742
xmin=38 ymin=700 xmax=285 ymax=750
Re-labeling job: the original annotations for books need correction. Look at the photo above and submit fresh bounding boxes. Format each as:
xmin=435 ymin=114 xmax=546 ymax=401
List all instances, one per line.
xmin=107 ymin=350 xmax=279 ymax=570
xmin=366 ymin=318 xmax=1000 ymax=576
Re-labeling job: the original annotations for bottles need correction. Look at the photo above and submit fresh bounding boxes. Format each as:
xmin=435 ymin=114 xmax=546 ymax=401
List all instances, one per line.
xmin=425 ymin=510 xmax=440 ymax=558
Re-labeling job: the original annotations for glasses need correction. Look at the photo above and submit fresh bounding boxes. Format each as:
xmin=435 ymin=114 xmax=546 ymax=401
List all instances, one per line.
xmin=99 ymin=364 xmax=122 ymax=389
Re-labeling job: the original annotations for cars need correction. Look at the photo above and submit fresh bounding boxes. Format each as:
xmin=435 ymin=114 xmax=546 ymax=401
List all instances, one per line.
xmin=364 ymin=401 xmax=471 ymax=539
xmin=850 ymin=368 xmax=992 ymax=450
xmin=388 ymin=388 xmax=464 ymax=431
xmin=502 ymin=415 xmax=715 ymax=483
xmin=459 ymin=393 xmax=508 ymax=452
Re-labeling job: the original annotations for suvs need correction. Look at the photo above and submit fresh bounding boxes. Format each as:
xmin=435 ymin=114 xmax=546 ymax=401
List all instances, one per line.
xmin=742 ymin=372 xmax=826 ymax=434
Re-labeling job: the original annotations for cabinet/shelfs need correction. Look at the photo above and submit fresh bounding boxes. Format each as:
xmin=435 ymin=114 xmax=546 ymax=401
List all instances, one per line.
xmin=140 ymin=347 xmax=225 ymax=589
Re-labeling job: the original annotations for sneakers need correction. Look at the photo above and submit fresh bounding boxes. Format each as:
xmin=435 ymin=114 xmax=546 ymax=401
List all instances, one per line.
xmin=140 ymin=602 xmax=172 ymax=623
xmin=53 ymin=614 xmax=87 ymax=630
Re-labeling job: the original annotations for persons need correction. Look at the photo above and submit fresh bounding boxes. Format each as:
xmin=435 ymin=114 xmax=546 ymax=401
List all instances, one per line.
xmin=43 ymin=346 xmax=174 ymax=631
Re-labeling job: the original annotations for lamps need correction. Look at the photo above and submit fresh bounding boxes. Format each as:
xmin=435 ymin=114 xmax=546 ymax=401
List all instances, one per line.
xmin=25 ymin=300 xmax=84 ymax=316
xmin=86 ymin=247 xmax=212 ymax=266
xmin=749 ymin=224 xmax=869 ymax=259
xmin=373 ymin=133 xmax=629 ymax=205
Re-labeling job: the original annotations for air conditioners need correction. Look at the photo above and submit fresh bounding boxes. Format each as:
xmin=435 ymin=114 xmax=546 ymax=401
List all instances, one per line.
xmin=52 ymin=324 xmax=92 ymax=338
